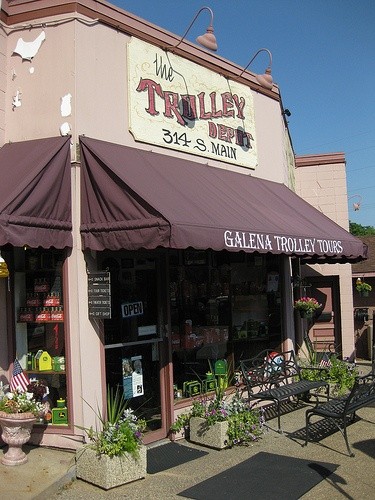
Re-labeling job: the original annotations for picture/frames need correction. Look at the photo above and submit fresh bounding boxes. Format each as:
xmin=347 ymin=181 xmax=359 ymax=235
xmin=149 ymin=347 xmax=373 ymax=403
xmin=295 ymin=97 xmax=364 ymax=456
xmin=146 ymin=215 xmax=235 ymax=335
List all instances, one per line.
xmin=114 ymin=248 xmax=264 ymax=322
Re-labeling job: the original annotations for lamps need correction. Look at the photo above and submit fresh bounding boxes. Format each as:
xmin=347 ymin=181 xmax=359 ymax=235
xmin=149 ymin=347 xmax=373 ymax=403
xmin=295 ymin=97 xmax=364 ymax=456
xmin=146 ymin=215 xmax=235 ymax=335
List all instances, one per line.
xmin=225 ymin=48 xmax=274 ymax=90
xmin=162 ymin=5 xmax=219 ymax=55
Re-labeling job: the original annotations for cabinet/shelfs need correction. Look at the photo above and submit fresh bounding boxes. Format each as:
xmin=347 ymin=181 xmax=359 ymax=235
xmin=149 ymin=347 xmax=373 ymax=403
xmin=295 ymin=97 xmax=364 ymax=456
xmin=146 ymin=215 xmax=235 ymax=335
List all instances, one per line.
xmin=14 ymin=317 xmax=68 ymax=428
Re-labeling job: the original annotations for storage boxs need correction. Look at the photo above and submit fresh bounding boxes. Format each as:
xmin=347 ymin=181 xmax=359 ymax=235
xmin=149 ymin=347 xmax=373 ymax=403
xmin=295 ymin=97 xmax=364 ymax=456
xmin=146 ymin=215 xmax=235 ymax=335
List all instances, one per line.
xmin=51 ymin=362 xmax=65 ymax=371
xmin=51 ymin=356 xmax=65 ymax=363
xmin=19 ymin=282 xmax=64 ymax=322
xmin=52 ymin=407 xmax=68 ymax=424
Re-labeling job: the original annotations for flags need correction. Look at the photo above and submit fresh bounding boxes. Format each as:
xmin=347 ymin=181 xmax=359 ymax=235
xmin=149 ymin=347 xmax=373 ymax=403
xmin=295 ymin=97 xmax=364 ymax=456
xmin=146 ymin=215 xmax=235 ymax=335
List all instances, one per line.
xmin=10 ymin=359 xmax=29 ymax=393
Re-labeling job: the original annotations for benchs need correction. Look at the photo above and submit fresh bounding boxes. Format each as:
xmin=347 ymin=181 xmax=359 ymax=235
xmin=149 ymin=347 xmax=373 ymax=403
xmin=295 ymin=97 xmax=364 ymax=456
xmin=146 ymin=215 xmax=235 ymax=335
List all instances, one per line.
xmin=237 ymin=347 xmax=331 ymax=434
xmin=302 ymin=370 xmax=375 ymax=456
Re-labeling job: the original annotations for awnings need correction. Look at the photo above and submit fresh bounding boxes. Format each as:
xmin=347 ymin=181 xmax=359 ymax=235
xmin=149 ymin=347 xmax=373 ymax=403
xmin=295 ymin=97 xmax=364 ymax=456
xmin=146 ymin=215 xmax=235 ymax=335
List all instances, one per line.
xmin=0 ymin=135 xmax=367 ymax=266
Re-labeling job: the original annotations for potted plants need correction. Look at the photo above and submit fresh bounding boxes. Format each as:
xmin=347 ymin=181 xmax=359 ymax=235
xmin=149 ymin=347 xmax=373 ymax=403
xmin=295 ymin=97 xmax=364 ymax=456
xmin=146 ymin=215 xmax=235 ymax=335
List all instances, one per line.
xmin=355 ymin=283 xmax=372 ymax=297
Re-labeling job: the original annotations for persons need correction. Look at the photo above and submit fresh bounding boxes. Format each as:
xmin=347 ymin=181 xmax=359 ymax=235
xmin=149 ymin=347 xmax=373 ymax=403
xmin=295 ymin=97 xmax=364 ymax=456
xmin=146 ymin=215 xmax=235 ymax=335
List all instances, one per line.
xmin=133 ymin=360 xmax=142 ymax=374
xmin=138 ymin=386 xmax=142 ymax=393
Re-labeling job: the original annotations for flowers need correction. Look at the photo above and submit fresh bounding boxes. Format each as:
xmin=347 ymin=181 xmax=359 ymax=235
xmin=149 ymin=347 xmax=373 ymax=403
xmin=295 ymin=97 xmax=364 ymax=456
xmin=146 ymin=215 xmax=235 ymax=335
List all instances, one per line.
xmin=169 ymin=397 xmax=270 ymax=454
xmin=291 ymin=295 xmax=323 ymax=310
xmin=1 ymin=384 xmax=47 ymax=415
xmin=291 ymin=333 xmax=363 ymax=398
xmin=74 ymin=379 xmax=161 ymax=455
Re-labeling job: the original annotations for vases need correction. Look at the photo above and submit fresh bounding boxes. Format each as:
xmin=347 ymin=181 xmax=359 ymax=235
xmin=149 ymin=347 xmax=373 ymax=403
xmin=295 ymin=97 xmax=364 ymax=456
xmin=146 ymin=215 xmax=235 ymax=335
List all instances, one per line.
xmin=299 ymin=308 xmax=314 ymax=319
xmin=308 ymin=376 xmax=351 ymax=401
xmin=189 ymin=415 xmax=235 ymax=450
xmin=75 ymin=441 xmax=148 ymax=489
xmin=0 ymin=410 xmax=40 ymax=466
